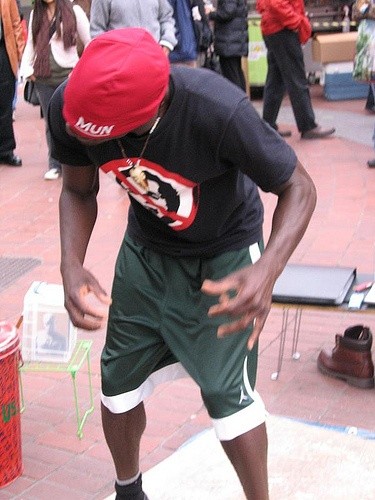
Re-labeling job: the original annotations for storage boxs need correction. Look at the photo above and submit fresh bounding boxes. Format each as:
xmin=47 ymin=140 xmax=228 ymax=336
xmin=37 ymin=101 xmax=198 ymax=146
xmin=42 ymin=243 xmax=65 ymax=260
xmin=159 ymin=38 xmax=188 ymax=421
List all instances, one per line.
xmin=20 ymin=280 xmax=76 ymax=363
xmin=313 ymin=32 xmax=358 ymax=62
xmin=320 ymin=64 xmax=369 ymax=100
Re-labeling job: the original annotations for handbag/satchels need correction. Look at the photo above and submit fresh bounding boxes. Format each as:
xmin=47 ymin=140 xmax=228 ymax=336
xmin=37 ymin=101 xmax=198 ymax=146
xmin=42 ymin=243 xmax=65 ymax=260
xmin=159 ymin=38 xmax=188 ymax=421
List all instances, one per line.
xmin=24 ymin=80 xmax=41 ymax=105
xmin=194 ymin=20 xmax=215 ymax=54
xmin=352 ymin=17 xmax=375 ymax=84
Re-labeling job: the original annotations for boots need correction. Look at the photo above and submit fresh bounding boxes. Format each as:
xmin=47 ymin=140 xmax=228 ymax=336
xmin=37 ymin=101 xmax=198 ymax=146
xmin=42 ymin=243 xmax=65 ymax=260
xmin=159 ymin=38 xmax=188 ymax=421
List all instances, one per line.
xmin=318 ymin=325 xmax=374 ymax=388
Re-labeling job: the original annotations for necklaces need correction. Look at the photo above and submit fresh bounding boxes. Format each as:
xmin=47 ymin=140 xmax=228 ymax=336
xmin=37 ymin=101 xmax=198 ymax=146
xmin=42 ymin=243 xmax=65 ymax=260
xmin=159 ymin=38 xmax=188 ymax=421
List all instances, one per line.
xmin=116 ymin=117 xmax=162 ymax=189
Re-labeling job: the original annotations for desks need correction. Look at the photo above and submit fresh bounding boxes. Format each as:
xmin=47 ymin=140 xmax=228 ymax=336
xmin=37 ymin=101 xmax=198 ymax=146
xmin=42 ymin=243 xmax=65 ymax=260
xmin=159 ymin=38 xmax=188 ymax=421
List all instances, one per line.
xmin=270 ymin=273 xmax=375 ymax=380
xmin=248 ymin=13 xmax=268 ymax=90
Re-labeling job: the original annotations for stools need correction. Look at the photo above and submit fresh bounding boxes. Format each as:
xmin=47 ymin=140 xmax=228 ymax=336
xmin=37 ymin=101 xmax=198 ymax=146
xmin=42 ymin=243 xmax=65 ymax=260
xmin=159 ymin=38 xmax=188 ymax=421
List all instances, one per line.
xmin=15 ymin=340 xmax=96 ymax=439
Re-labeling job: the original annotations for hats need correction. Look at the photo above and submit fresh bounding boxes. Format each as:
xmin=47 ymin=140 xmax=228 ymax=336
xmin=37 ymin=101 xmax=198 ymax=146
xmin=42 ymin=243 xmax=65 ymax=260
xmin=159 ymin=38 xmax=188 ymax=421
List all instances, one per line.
xmin=63 ymin=28 xmax=170 ymax=138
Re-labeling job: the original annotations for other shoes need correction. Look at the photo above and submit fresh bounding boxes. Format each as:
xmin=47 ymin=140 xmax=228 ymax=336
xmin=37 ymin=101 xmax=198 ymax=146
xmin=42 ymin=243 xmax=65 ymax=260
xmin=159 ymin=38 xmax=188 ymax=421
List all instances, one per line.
xmin=302 ymin=125 xmax=336 ymax=139
xmin=368 ymin=160 xmax=375 ymax=167
xmin=0 ymin=154 xmax=21 ymax=166
xmin=45 ymin=168 xmax=62 ymax=180
xmin=277 ymin=128 xmax=292 ymax=136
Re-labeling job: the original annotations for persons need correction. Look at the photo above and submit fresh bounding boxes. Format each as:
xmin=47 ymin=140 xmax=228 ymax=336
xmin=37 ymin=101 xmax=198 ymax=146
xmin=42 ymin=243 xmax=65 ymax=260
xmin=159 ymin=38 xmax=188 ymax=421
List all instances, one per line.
xmin=19 ymin=0 xmax=91 ymax=179
xmin=47 ymin=28 xmax=317 ymax=499
xmin=88 ymin=0 xmax=178 ymax=56
xmin=0 ymin=0 xmax=25 ymax=165
xmin=255 ymin=1 xmax=336 ymax=140
xmin=168 ymin=0 xmax=196 ymax=68
xmin=204 ymin=0 xmax=249 ymax=92
xmin=352 ymin=0 xmax=375 ymax=166
